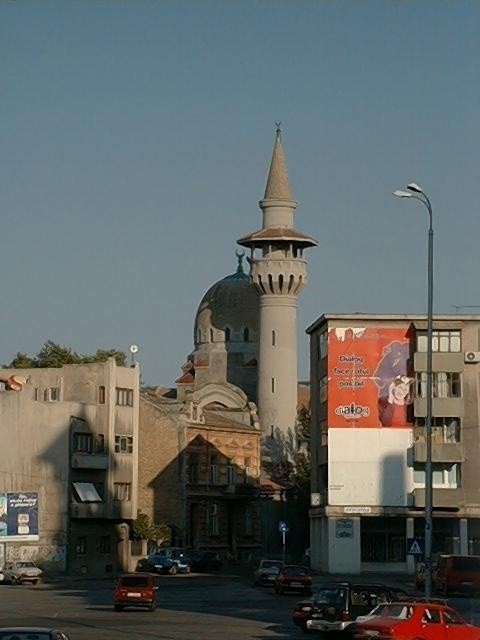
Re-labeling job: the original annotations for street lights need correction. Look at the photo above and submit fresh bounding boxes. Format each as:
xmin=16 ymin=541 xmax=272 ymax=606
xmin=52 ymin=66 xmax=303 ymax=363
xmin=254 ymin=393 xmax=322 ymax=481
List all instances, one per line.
xmin=394 ymin=180 xmax=435 ymax=599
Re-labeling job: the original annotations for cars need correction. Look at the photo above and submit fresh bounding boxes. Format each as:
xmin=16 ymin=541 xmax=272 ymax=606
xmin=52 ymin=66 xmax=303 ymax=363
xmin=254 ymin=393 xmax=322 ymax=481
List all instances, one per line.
xmin=2 ymin=560 xmax=43 ymax=586
xmin=146 ymin=546 xmax=203 ymax=575
xmin=0 ymin=627 xmax=71 ymax=640
xmin=112 ymin=573 xmax=161 ymax=613
xmin=253 ymin=558 xmax=313 ymax=593
xmin=293 ymin=582 xmax=479 ymax=640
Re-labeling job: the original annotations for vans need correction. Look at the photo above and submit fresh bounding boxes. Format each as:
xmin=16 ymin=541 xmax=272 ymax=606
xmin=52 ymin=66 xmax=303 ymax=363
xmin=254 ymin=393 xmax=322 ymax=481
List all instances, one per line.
xmin=437 ymin=552 xmax=480 ymax=593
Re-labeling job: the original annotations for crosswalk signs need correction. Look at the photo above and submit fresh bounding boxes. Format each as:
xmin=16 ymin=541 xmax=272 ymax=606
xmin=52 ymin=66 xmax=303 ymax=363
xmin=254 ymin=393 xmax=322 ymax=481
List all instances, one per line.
xmin=407 ymin=539 xmax=425 ymax=554
